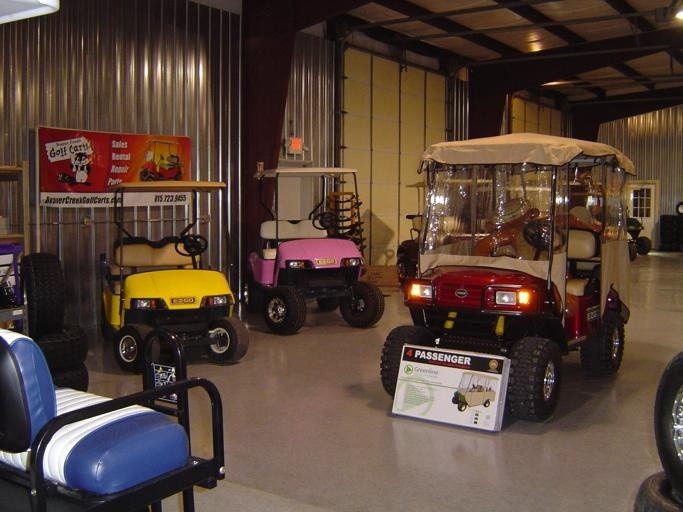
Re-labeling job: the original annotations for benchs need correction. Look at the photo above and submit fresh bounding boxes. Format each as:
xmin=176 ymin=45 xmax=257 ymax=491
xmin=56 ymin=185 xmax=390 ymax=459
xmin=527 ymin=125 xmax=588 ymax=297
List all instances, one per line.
xmin=260 ymin=218 xmax=328 ymax=259
xmin=513 ymin=228 xmax=600 ymax=297
xmin=109 ymin=235 xmax=201 ymax=296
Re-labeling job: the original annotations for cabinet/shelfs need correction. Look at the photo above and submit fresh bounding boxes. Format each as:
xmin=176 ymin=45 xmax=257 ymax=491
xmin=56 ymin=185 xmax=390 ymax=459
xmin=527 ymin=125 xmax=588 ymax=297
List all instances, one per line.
xmin=0 ymin=159 xmax=31 ymax=336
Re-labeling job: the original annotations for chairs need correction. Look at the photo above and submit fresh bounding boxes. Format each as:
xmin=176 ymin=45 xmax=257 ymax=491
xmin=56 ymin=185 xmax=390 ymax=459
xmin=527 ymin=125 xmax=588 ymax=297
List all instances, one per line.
xmin=0 ymin=330 xmax=228 ymax=512
